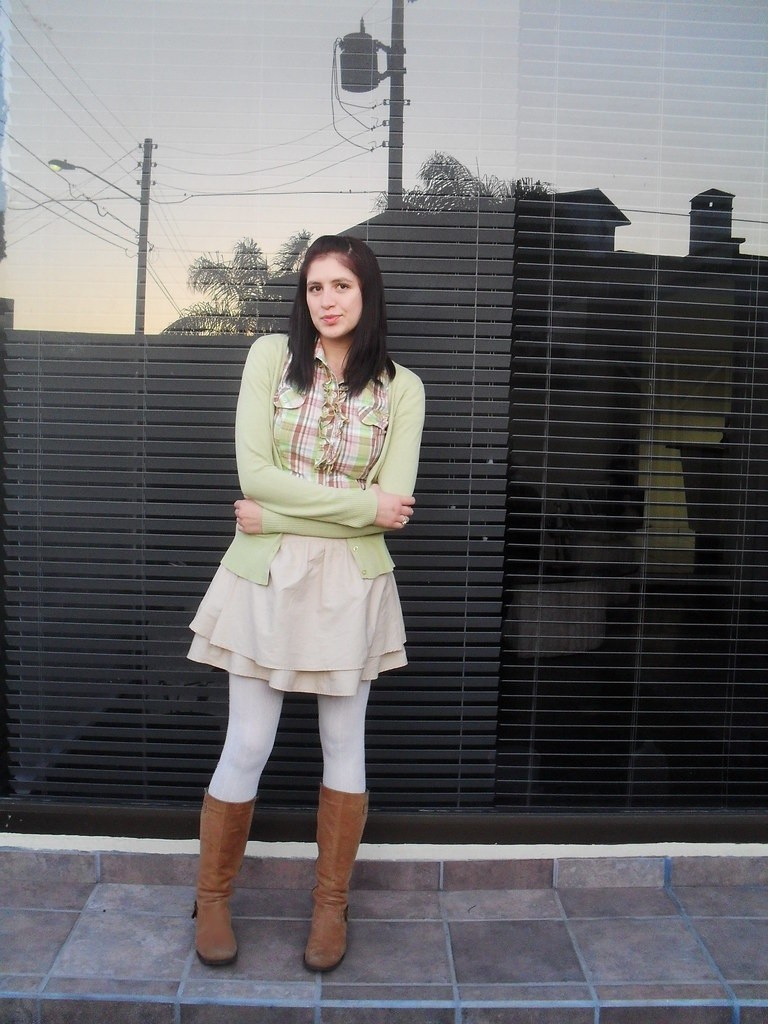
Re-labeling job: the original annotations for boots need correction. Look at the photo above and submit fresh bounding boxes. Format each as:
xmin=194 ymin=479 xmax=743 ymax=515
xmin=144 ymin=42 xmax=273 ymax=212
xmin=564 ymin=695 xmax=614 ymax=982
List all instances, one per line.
xmin=192 ymin=787 xmax=257 ymax=965
xmin=303 ymin=782 xmax=370 ymax=973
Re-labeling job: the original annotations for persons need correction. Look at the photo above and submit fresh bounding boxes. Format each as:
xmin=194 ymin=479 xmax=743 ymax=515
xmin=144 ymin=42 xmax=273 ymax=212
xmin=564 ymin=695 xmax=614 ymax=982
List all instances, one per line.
xmin=187 ymin=235 xmax=426 ymax=972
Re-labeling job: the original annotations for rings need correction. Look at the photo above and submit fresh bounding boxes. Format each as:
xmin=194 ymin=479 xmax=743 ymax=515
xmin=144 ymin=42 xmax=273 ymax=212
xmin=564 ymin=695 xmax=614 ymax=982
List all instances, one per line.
xmin=401 ymin=515 xmax=407 ymax=525
